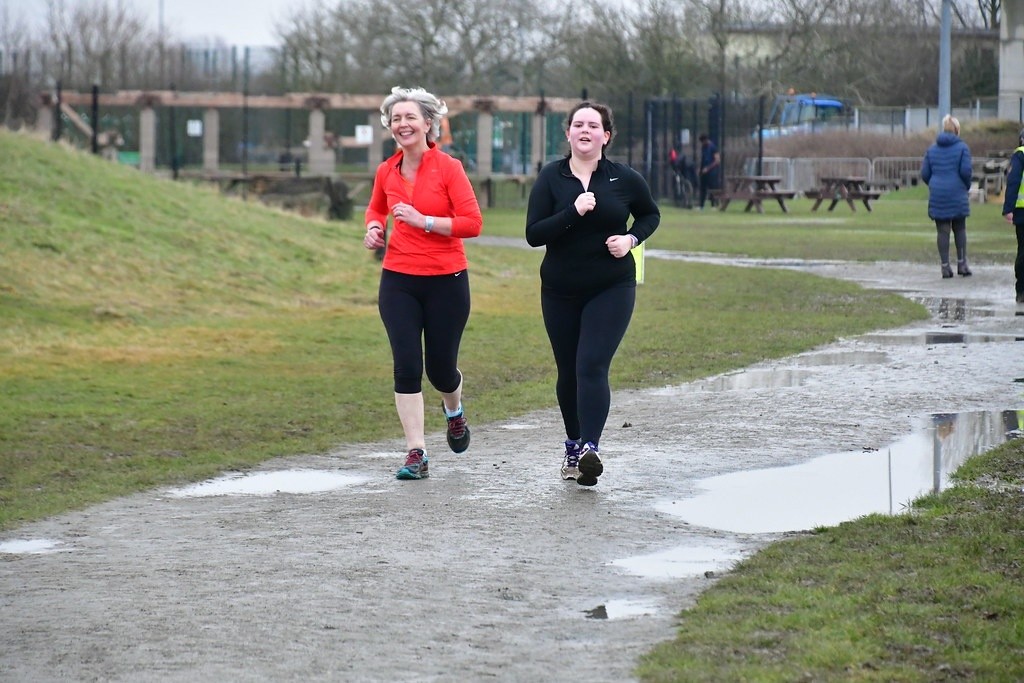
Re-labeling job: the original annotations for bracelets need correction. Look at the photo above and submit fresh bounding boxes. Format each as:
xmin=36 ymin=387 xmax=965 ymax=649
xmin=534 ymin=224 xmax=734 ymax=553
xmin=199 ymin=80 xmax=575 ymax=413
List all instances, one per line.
xmin=627 ymin=234 xmax=638 ymax=249
xmin=425 ymin=215 xmax=434 ymax=233
xmin=369 ymin=226 xmax=381 ymax=230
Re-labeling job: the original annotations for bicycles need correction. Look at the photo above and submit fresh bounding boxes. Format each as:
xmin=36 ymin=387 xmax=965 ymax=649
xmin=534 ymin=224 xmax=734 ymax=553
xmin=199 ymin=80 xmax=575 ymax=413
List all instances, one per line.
xmin=667 ymin=153 xmax=695 ymax=209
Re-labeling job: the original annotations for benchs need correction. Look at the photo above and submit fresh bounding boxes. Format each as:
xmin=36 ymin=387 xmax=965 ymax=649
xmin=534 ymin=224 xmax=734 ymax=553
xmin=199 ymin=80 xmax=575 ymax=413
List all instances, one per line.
xmin=805 ymin=177 xmax=880 ymax=212
xmin=713 ymin=176 xmax=793 ymax=213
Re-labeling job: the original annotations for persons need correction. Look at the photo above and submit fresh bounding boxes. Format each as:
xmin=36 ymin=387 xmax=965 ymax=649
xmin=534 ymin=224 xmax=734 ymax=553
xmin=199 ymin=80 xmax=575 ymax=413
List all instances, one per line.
xmin=364 ymin=86 xmax=482 ymax=481
xmin=1002 ymin=130 xmax=1024 ymax=302
xmin=695 ymin=133 xmax=721 ymax=211
xmin=921 ymin=113 xmax=974 ymax=280
xmin=525 ymin=100 xmax=661 ymax=486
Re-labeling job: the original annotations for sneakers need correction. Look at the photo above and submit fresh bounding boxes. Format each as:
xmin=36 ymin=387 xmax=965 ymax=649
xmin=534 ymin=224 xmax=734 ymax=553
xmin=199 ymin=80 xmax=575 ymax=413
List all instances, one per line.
xmin=442 ymin=398 xmax=470 ymax=453
xmin=397 ymin=449 xmax=429 ymax=480
xmin=561 ymin=440 xmax=581 ymax=480
xmin=577 ymin=442 xmax=602 ymax=486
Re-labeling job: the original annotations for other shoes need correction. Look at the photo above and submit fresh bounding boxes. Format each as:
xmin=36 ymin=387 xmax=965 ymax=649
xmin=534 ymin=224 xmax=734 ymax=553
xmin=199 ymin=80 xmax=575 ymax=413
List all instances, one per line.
xmin=1015 ymin=297 xmax=1024 ymax=316
xmin=942 ymin=263 xmax=953 ymax=278
xmin=957 ymin=261 xmax=971 ymax=276
